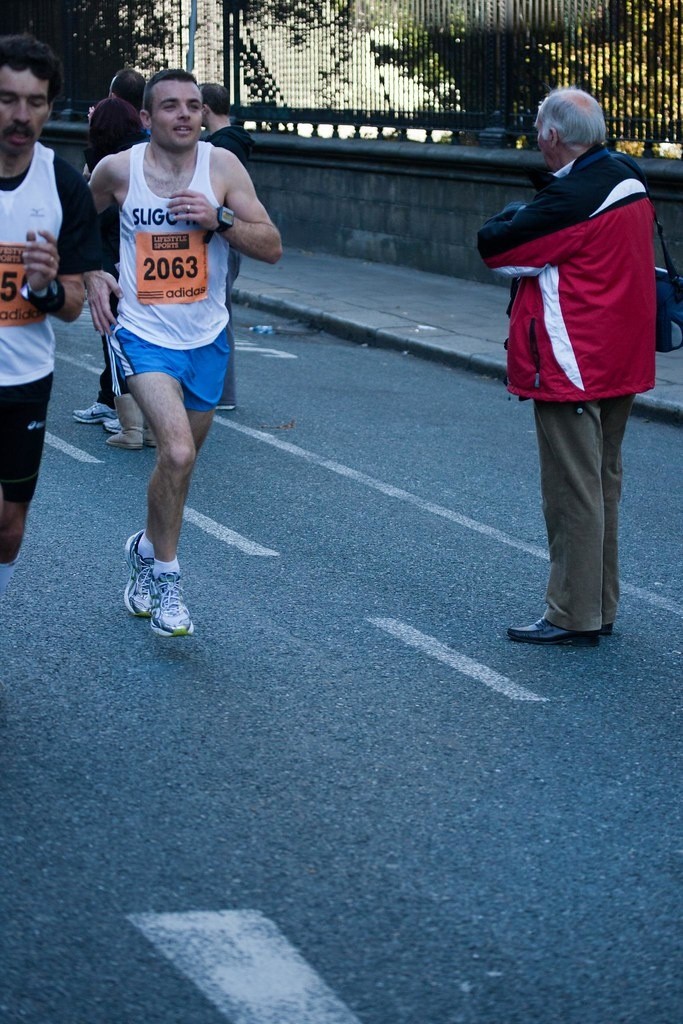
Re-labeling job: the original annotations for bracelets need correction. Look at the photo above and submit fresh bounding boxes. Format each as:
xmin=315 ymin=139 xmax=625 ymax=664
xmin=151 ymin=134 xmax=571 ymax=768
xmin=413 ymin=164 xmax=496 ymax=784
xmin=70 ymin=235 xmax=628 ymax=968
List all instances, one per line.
xmin=22 ymin=278 xmax=65 ymax=314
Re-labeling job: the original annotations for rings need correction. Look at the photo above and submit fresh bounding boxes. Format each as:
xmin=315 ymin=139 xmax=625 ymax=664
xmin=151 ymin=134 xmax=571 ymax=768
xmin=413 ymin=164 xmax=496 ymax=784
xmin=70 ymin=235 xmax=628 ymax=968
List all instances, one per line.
xmin=49 ymin=256 xmax=54 ymax=262
xmin=188 ymin=205 xmax=190 ymax=211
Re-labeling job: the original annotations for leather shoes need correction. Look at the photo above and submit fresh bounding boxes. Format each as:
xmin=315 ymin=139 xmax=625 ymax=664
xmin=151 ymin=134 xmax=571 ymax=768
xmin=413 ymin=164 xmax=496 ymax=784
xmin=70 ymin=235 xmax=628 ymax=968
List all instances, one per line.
xmin=506 ymin=617 xmax=600 ymax=647
xmin=600 ymin=621 xmax=613 ymax=634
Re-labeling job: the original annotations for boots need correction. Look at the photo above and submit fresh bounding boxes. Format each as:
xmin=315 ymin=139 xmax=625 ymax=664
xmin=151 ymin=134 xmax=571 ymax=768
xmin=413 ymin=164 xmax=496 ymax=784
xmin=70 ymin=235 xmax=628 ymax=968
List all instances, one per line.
xmin=105 ymin=391 xmax=144 ymax=449
xmin=142 ymin=411 xmax=156 ymax=447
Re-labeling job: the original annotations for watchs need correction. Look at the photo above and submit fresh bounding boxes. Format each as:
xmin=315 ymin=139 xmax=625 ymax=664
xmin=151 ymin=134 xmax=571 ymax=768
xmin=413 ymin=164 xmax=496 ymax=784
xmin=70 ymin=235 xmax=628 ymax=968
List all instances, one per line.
xmin=202 ymin=207 xmax=234 ymax=244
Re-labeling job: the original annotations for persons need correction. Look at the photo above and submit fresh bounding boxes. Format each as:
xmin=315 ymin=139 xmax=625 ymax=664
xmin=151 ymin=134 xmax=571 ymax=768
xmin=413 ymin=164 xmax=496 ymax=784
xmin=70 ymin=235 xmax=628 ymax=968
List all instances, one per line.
xmin=88 ymin=69 xmax=283 ymax=638
xmin=475 ymin=87 xmax=656 ymax=648
xmin=198 ymin=83 xmax=255 ymax=414
xmin=0 ymin=35 xmax=107 ymax=599
xmin=74 ymin=70 xmax=153 ymax=449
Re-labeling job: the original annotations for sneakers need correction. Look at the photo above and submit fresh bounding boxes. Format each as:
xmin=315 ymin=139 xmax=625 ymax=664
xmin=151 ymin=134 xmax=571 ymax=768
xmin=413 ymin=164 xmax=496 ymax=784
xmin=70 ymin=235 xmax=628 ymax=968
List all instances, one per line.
xmin=72 ymin=403 xmax=117 ymax=423
xmin=103 ymin=418 xmax=123 ymax=433
xmin=124 ymin=529 xmax=153 ymax=616
xmin=148 ymin=570 xmax=195 ymax=636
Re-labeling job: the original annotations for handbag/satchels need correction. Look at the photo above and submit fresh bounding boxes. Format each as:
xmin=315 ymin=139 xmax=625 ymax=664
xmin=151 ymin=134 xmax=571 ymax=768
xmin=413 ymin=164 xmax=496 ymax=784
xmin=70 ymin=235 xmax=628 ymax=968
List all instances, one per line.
xmin=612 ymin=154 xmax=683 ymax=352
